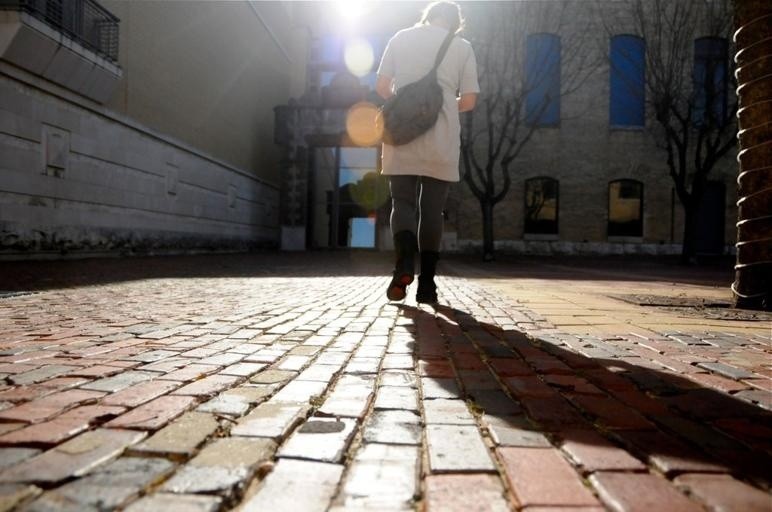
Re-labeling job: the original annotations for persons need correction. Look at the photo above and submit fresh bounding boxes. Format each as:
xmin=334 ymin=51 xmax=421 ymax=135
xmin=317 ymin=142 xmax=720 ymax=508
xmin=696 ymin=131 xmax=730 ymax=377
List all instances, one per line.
xmin=372 ymin=1 xmax=481 ymax=305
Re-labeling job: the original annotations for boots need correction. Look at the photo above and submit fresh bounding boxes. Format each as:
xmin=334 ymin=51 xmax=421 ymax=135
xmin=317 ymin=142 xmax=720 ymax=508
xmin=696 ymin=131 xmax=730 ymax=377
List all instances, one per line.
xmin=387 ymin=230 xmax=415 ymax=300
xmin=416 ymin=249 xmax=439 ymax=303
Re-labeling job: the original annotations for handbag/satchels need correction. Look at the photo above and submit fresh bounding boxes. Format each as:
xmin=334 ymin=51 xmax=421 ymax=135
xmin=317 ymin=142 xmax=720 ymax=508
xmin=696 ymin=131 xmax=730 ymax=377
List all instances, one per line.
xmin=375 ymin=75 xmax=442 ymax=144
